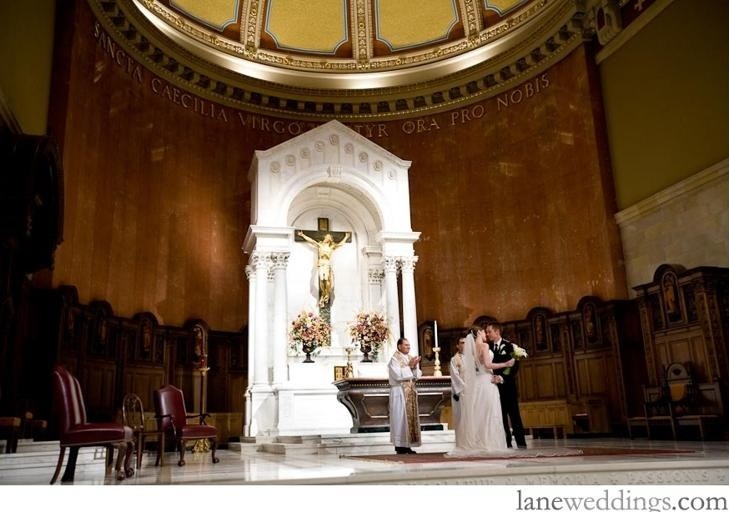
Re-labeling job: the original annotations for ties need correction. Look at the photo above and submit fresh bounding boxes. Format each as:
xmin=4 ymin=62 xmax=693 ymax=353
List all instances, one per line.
xmin=495 ymin=344 xmax=498 ymax=352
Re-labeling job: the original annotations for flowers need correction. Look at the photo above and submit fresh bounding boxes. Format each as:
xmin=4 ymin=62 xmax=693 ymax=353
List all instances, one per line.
xmin=348 ymin=308 xmax=393 ymax=353
xmin=288 ymin=308 xmax=331 ymax=358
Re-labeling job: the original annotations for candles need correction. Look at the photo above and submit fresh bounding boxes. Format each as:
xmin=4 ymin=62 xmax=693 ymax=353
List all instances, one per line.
xmin=432 ymin=318 xmax=439 ymax=347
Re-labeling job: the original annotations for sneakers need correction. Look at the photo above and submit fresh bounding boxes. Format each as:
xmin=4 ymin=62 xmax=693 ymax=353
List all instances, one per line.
xmin=394 ymin=447 xmax=417 ymax=455
xmin=516 ymin=442 xmax=528 ymax=449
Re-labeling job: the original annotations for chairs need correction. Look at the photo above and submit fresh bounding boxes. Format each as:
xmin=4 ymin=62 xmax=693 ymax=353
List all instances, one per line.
xmin=48 ymin=363 xmax=137 ymax=486
xmin=644 ymin=360 xmax=696 ymax=442
xmin=118 ymin=389 xmax=164 ymax=469
xmin=676 ymin=383 xmax=726 ymax=442
xmin=625 ymin=389 xmax=664 ymax=441
xmin=153 ymin=383 xmax=224 ymax=469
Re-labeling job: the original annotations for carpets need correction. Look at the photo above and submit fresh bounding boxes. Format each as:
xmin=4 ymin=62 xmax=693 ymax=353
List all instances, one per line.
xmin=340 ymin=446 xmax=696 ymax=464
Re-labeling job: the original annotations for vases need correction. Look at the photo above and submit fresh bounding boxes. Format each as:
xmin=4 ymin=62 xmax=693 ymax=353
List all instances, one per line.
xmin=359 ymin=347 xmax=374 ymax=363
xmin=302 ymin=345 xmax=316 ymax=364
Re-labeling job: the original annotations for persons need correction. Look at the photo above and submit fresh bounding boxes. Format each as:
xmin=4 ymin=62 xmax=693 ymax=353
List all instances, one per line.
xmin=486 ymin=321 xmax=527 ymax=448
xmin=449 ymin=334 xmax=467 ymax=430
xmin=456 ymin=326 xmax=514 ymax=452
xmin=387 ymin=338 xmax=422 ymax=454
xmin=297 ymin=230 xmax=351 ymax=305
